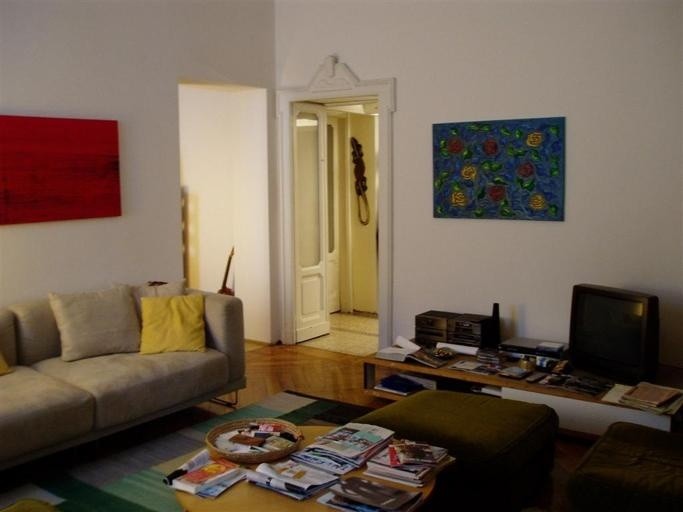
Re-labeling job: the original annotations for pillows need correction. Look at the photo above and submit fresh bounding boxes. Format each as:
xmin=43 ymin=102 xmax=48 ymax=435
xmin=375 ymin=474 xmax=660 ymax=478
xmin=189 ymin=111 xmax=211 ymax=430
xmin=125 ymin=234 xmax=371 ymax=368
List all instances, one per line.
xmin=140 ymin=294 xmax=205 ymax=354
xmin=131 ymin=278 xmax=186 ymax=319
xmin=0 ymin=355 xmax=14 ymax=375
xmin=48 ymin=284 xmax=140 ymax=361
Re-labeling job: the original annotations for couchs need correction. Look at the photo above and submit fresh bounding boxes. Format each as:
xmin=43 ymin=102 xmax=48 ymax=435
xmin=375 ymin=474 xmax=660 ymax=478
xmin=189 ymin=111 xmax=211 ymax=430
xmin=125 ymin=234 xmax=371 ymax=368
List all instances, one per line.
xmin=1 ymin=288 xmax=246 ymax=471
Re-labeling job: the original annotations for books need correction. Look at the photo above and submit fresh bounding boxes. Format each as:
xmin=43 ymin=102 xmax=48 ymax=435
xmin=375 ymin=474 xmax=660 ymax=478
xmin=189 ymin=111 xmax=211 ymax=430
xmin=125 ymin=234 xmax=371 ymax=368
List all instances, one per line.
xmin=246 ymin=459 xmax=340 ymax=500
xmin=162 ymin=449 xmax=247 ymax=500
xmin=447 ymin=348 xmax=508 ymax=375
xmin=374 ymin=376 xmax=424 ymax=396
xmin=496 ymin=367 xmax=532 ymax=380
xmin=618 ymin=381 xmax=683 ymax=416
xmin=374 ymin=335 xmax=457 ymax=367
xmin=364 ymin=437 xmax=457 ymax=488
xmin=539 ymin=373 xmax=604 ymax=397
xmin=289 ymin=422 xmax=396 ymax=475
xmin=316 ymin=476 xmax=423 ymax=512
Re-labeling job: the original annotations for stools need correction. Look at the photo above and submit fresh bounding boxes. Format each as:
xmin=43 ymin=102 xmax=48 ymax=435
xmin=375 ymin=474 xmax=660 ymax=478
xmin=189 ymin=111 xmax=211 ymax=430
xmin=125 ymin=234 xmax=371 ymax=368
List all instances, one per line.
xmin=354 ymin=389 xmax=558 ymax=511
xmin=566 ymin=422 xmax=682 ymax=511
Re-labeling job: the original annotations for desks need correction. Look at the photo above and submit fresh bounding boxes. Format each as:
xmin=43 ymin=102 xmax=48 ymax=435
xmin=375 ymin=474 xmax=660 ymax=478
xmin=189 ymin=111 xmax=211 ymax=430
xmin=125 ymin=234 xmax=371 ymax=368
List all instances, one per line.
xmin=360 ymin=353 xmax=683 ymax=436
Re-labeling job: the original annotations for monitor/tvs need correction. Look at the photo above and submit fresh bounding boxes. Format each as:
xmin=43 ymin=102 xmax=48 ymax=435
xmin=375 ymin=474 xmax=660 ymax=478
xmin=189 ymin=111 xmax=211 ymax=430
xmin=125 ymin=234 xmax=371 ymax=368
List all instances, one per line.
xmin=568 ymin=283 xmax=659 ymax=385
